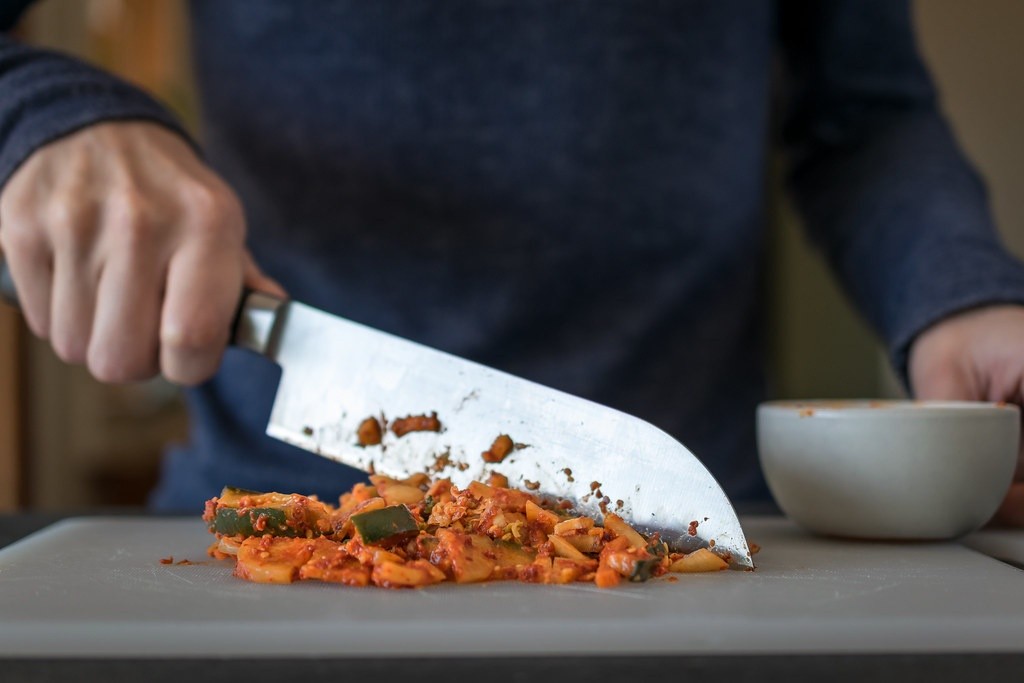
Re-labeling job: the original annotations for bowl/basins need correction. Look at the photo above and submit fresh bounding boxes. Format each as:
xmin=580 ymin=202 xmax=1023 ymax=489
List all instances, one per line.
xmin=759 ymin=399 xmax=1019 ymax=543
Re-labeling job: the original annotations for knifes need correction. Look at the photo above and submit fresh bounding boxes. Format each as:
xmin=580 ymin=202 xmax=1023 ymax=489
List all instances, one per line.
xmin=0 ymin=258 xmax=758 ymax=572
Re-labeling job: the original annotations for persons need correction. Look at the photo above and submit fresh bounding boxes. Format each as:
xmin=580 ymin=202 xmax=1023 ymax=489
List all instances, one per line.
xmin=0 ymin=0 xmax=1024 ymax=520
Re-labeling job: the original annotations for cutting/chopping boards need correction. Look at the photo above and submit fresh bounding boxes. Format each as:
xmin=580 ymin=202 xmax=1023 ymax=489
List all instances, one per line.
xmin=0 ymin=518 xmax=1024 ymax=672
